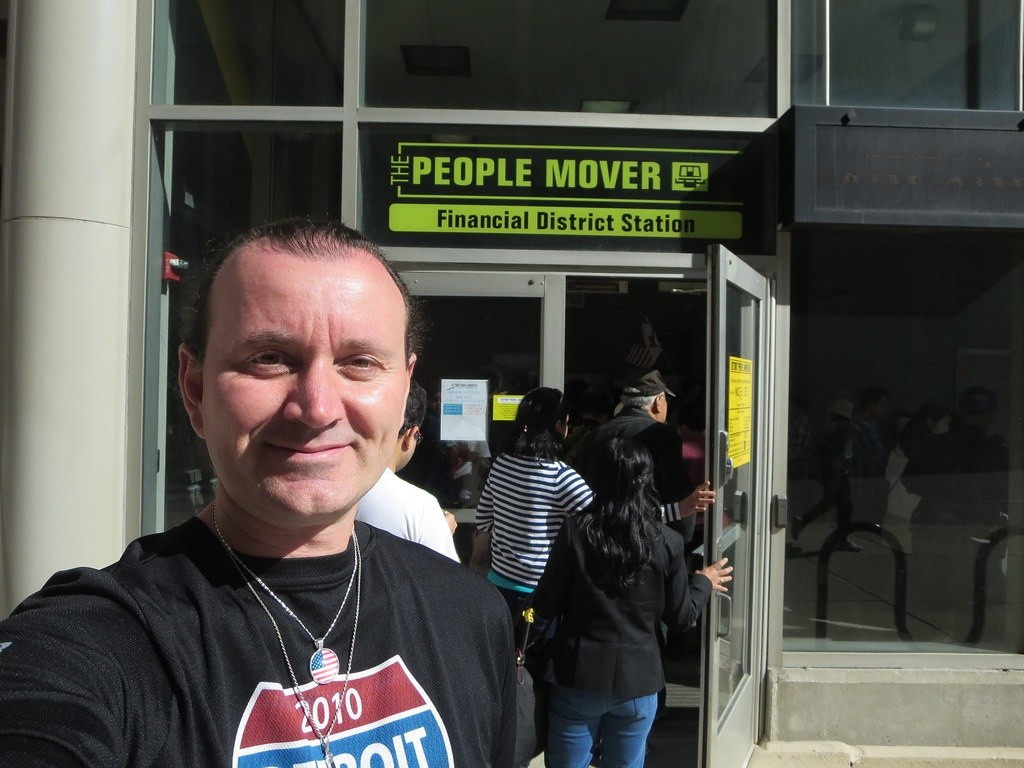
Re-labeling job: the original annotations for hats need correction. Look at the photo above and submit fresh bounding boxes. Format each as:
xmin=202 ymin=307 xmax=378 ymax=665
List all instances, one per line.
xmin=621 ymin=368 xmax=677 ymax=397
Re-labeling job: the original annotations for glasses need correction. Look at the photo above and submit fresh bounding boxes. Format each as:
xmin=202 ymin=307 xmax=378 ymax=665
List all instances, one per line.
xmin=416 ymin=430 xmax=422 ymax=444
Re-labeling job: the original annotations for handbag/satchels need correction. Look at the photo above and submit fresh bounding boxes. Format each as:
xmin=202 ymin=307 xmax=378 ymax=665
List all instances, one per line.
xmin=514 ymin=608 xmax=547 ymax=768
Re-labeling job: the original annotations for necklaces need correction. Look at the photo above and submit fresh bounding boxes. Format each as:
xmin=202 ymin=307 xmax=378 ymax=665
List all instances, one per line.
xmin=209 ymin=494 xmax=364 ymax=768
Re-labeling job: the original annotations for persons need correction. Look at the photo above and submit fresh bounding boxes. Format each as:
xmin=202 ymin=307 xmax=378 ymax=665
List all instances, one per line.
xmin=788 ymin=365 xmax=901 ymax=555
xmin=423 ymin=363 xmax=535 ymax=507
xmin=522 ymin=432 xmax=736 ymax=768
xmin=474 ymin=386 xmax=717 ymax=675
xmin=353 ymin=376 xmax=460 ymax=567
xmin=568 ymin=367 xmax=683 ymax=532
xmin=790 ymin=384 xmax=1008 ymax=643
xmin=1 ymin=211 xmax=540 ymax=768
